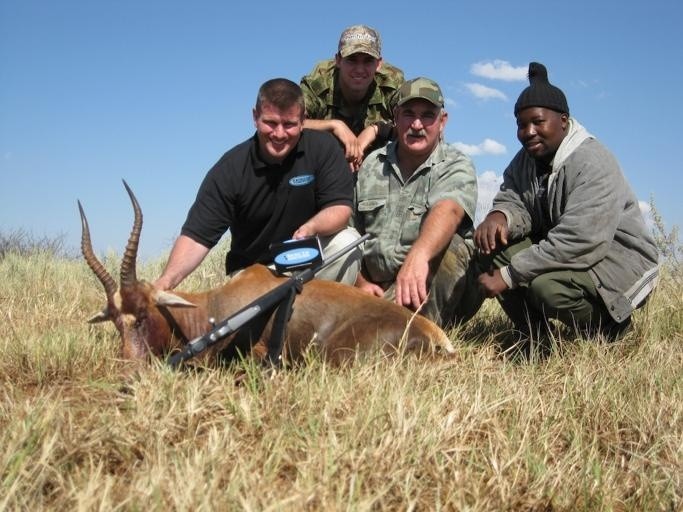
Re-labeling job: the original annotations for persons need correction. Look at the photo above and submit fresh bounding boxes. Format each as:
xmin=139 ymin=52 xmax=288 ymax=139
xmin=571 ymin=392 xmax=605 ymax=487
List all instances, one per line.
xmin=471 ymin=60 xmax=660 ymax=358
xmin=344 ymin=76 xmax=489 ymax=331
xmin=295 ymin=26 xmax=406 ymax=236
xmin=150 ymin=79 xmax=364 ymax=288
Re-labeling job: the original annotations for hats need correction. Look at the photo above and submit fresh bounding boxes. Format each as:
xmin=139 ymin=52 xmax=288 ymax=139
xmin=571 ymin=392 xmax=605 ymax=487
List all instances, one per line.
xmin=514 ymin=62 xmax=568 ymax=115
xmin=338 ymin=24 xmax=382 ymax=61
xmin=398 ymin=77 xmax=445 ymax=108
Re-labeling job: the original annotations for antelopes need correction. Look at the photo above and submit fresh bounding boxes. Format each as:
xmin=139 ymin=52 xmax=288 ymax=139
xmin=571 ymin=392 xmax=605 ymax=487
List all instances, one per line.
xmin=77 ymin=179 xmax=455 ymax=384
